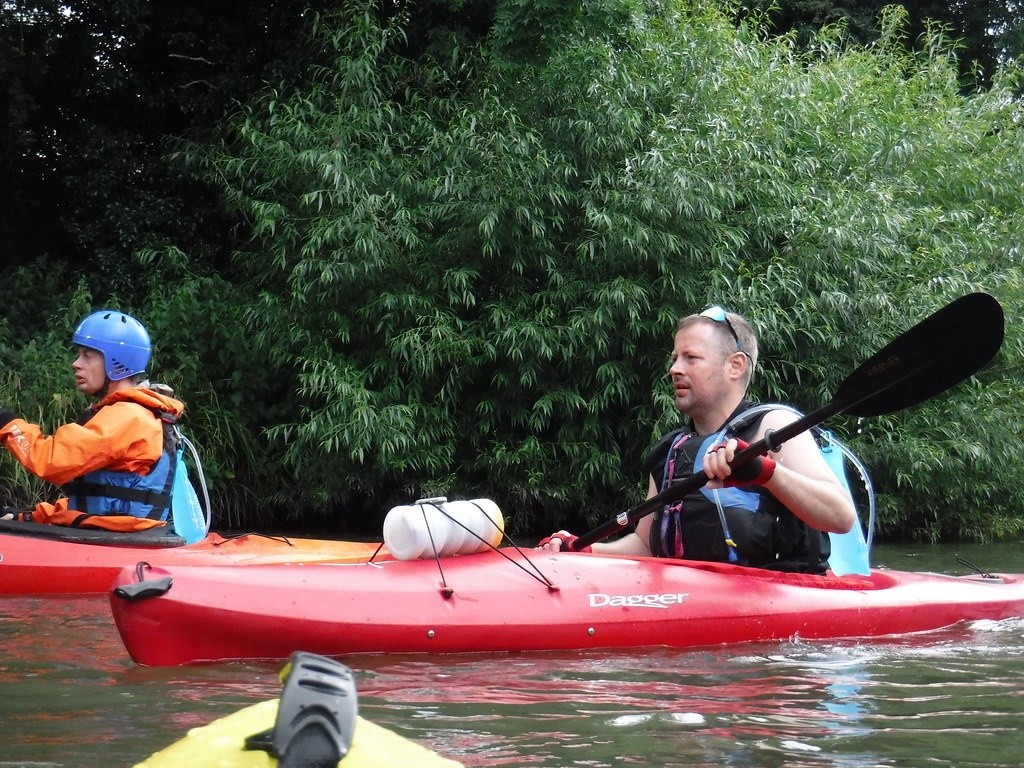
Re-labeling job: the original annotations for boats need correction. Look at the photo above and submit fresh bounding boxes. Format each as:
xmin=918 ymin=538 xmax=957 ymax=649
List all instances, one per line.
xmin=0 ymin=514 xmax=391 ymax=597
xmin=109 ymin=497 xmax=1024 ymax=665
xmin=131 ymin=651 xmax=466 ymax=768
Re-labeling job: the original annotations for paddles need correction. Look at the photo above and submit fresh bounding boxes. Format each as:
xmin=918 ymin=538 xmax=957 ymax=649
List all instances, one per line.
xmin=558 ymin=292 xmax=1005 ymax=552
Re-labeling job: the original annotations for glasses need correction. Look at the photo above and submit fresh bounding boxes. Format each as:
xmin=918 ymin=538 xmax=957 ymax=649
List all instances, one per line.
xmin=700 ymin=305 xmax=743 ymax=354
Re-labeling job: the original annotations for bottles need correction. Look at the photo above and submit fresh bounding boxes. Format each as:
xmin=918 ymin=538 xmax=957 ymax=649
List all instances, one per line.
xmin=817 ymin=444 xmax=870 ymax=578
xmin=171 ymin=450 xmax=206 ymax=543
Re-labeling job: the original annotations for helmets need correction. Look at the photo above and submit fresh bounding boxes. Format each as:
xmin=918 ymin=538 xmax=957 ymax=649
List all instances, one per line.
xmin=74 ymin=310 xmax=151 ymax=379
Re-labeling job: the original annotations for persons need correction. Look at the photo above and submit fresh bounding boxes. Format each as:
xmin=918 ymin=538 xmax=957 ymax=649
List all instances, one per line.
xmin=0 ymin=311 xmax=184 ymax=533
xmin=534 ymin=303 xmax=855 ymax=577
